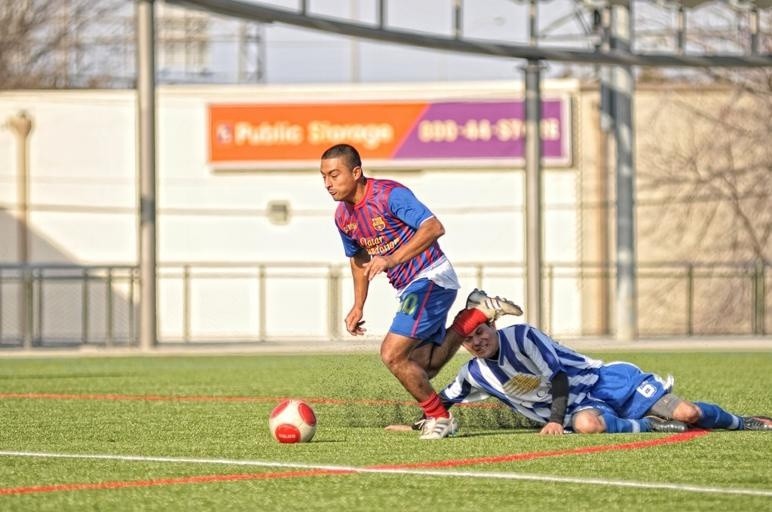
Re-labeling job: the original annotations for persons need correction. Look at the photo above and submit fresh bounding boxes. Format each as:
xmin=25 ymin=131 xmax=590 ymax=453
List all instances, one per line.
xmin=383 ymin=306 xmax=771 ymax=437
xmin=319 ymin=143 xmax=527 ymax=441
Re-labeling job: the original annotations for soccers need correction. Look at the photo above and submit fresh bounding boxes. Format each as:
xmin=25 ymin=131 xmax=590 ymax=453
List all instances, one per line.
xmin=269 ymin=401 xmax=316 ymax=444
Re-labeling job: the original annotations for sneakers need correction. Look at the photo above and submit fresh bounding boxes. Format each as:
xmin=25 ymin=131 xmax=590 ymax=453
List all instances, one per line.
xmin=643 ymin=414 xmax=689 ymax=433
xmin=414 ymin=410 xmax=460 ymax=440
xmin=740 ymin=416 xmax=772 ymax=430
xmin=466 ymin=288 xmax=523 ymax=326
